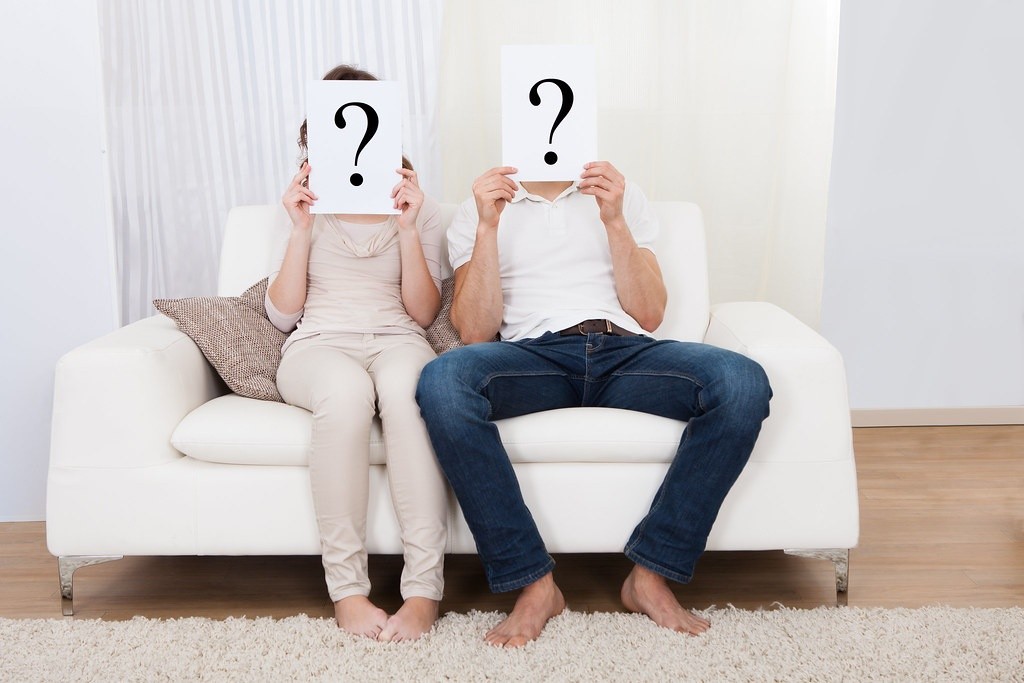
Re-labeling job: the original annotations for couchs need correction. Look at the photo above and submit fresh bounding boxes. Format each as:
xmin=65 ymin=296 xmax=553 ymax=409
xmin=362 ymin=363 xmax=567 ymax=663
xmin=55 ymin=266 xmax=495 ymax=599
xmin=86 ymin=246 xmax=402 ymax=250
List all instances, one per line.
xmin=45 ymin=207 xmax=860 ymax=619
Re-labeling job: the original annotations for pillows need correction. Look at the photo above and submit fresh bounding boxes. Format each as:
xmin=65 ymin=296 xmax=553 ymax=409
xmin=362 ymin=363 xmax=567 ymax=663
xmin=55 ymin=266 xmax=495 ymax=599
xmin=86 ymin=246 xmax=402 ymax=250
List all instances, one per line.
xmin=422 ymin=274 xmax=502 ymax=358
xmin=151 ymin=278 xmax=293 ymax=404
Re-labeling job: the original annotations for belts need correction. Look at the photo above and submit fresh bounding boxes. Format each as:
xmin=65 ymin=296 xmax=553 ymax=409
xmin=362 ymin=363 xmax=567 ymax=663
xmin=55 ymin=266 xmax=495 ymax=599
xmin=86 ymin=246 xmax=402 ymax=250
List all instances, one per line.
xmin=560 ymin=319 xmax=641 ymax=337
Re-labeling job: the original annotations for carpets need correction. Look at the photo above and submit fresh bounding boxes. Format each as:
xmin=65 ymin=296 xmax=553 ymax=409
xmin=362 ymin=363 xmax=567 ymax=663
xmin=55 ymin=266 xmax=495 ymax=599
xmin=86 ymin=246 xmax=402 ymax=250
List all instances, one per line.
xmin=0 ymin=604 xmax=1023 ymax=682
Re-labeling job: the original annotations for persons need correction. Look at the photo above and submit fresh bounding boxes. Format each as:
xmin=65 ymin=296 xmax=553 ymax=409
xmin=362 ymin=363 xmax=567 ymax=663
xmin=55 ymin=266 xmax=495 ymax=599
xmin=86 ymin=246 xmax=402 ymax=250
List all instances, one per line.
xmin=264 ymin=62 xmax=455 ymax=648
xmin=416 ymin=60 xmax=773 ymax=644
